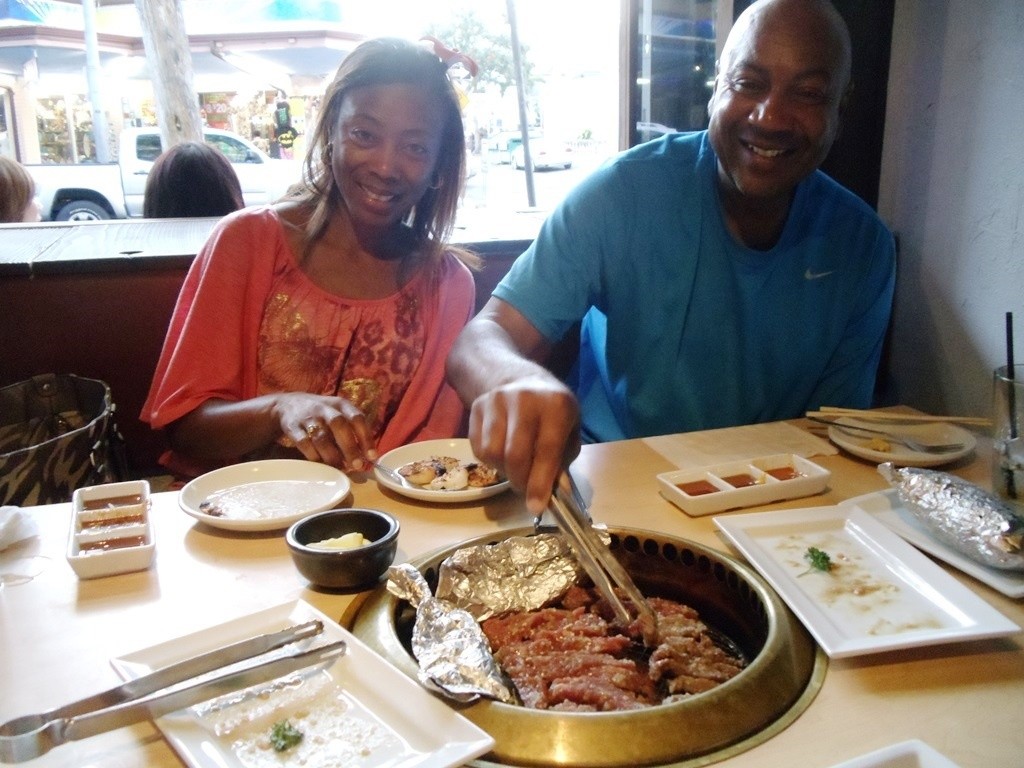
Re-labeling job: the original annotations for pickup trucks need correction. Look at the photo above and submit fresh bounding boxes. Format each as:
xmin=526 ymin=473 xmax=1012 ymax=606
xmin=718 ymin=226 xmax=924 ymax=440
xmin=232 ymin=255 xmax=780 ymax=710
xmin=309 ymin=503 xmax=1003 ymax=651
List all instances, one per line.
xmin=18 ymin=126 xmax=303 ymax=221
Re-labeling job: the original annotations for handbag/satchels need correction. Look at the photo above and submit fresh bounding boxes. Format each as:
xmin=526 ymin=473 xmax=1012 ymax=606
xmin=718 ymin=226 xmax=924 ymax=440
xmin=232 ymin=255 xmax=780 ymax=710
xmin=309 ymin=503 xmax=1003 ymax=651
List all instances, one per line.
xmin=0 ymin=373 xmax=127 ymax=505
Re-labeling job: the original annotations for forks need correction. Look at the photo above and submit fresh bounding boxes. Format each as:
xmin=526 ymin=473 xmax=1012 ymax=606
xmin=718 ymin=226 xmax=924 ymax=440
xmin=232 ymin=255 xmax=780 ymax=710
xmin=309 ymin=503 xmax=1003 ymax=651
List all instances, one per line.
xmin=807 ymin=415 xmax=965 ymax=454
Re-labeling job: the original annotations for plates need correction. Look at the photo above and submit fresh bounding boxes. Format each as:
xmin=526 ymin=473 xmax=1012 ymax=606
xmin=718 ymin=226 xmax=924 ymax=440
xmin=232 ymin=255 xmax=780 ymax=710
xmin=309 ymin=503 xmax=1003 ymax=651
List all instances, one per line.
xmin=837 ymin=486 xmax=1024 ymax=599
xmin=107 ymin=597 xmax=497 ymax=768
xmin=178 ymin=458 xmax=351 ymax=533
xmin=828 ymin=412 xmax=978 ymax=467
xmin=713 ymin=502 xmax=1024 ymax=660
xmin=373 ymin=437 xmax=512 ymax=504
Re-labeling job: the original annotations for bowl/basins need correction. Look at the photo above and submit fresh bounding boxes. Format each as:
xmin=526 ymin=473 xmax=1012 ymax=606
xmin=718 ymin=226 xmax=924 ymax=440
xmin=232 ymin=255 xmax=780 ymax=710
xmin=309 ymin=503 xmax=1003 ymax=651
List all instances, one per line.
xmin=829 ymin=738 xmax=964 ymax=768
xmin=658 ymin=453 xmax=832 ymax=518
xmin=66 ymin=480 xmax=156 ymax=581
xmin=284 ymin=507 xmax=401 ymax=590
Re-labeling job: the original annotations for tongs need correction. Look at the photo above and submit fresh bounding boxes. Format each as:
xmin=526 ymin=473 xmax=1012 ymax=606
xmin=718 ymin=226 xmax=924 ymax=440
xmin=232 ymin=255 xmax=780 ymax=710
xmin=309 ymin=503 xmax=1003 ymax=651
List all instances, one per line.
xmin=541 ymin=482 xmax=663 ymax=628
xmin=0 ymin=619 xmax=348 ymax=764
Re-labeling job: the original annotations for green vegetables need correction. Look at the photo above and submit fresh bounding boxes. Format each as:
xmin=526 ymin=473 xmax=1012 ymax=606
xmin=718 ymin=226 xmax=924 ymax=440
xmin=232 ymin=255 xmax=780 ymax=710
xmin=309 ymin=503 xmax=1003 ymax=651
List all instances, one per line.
xmin=268 ymin=719 xmax=303 ymax=751
xmin=796 ymin=547 xmax=831 ymax=576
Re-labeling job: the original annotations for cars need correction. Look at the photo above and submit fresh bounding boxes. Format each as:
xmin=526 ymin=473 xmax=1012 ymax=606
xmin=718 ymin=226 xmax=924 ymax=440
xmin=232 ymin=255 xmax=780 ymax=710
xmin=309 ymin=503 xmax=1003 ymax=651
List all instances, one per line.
xmin=486 ymin=131 xmax=574 ymax=171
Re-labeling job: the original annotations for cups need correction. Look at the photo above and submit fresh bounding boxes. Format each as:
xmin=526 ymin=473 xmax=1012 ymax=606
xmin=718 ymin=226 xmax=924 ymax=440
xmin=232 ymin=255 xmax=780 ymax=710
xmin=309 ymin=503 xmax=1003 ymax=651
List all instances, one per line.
xmin=990 ymin=363 xmax=1024 ymax=506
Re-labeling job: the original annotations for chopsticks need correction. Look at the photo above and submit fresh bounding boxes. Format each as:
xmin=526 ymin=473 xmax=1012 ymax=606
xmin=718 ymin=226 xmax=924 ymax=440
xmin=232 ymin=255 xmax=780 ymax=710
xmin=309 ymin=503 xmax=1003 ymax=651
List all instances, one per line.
xmin=805 ymin=406 xmax=993 ymax=426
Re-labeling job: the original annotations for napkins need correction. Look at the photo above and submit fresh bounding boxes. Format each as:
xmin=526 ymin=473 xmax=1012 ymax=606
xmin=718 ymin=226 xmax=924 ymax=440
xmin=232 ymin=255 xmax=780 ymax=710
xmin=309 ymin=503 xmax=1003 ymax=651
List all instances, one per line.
xmin=0 ymin=505 xmax=40 ymax=552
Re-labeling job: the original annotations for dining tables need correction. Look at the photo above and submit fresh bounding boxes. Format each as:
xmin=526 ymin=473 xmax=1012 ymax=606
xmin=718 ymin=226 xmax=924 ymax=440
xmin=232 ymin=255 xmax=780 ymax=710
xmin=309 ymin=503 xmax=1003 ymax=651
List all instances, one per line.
xmin=0 ymin=405 xmax=1024 ymax=768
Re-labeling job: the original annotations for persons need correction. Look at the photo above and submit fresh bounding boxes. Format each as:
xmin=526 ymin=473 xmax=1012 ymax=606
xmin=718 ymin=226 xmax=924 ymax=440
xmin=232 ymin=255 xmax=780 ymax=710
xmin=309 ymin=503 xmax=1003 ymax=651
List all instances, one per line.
xmin=0 ymin=114 xmax=281 ymax=226
xmin=443 ymin=0 xmax=900 ymax=510
xmin=140 ymin=38 xmax=481 ymax=486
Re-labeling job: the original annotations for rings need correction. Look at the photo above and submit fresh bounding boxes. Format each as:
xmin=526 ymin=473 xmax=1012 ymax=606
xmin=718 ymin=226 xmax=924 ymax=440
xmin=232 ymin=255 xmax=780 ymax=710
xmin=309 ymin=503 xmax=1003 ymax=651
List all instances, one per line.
xmin=306 ymin=424 xmax=324 ymax=438
